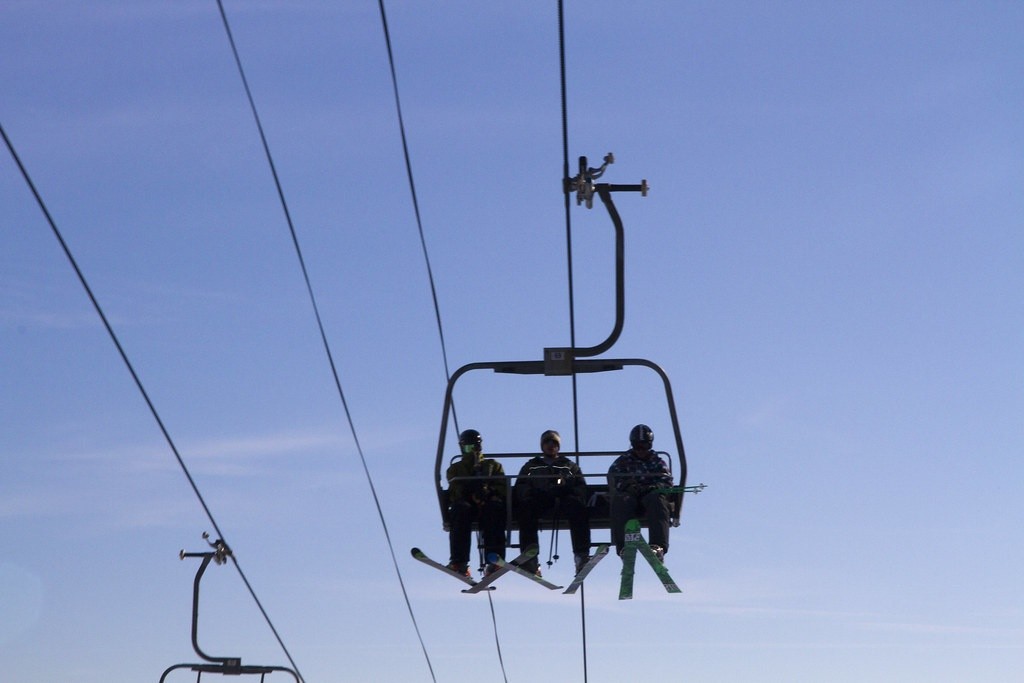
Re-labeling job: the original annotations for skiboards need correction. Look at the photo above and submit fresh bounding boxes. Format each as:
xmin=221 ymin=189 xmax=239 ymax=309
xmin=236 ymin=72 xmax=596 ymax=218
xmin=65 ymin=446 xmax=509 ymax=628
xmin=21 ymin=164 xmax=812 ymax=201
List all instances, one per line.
xmin=488 ymin=544 xmax=609 ymax=594
xmin=619 ymin=519 xmax=682 ymax=600
xmin=411 ymin=543 xmax=539 ymax=593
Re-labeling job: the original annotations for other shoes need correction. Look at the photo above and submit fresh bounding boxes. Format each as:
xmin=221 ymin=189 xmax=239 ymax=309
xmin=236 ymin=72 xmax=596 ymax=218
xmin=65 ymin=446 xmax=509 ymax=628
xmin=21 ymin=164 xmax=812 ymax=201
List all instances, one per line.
xmin=448 ymin=562 xmax=469 ymax=576
xmin=649 ymin=544 xmax=665 ymax=563
xmin=485 ymin=564 xmax=499 ymax=574
xmin=574 ymin=554 xmax=588 ymax=571
xmin=520 ymin=559 xmax=538 ymax=574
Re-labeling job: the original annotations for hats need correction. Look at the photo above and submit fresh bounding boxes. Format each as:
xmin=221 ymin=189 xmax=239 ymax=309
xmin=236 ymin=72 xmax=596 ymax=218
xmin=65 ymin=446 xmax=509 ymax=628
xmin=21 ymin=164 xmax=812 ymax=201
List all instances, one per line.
xmin=540 ymin=430 xmax=561 ymax=449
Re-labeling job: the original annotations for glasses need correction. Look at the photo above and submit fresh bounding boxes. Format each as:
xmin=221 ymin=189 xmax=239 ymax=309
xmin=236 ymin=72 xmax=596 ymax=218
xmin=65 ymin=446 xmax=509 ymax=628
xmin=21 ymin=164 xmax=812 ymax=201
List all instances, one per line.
xmin=633 ymin=441 xmax=653 ymax=450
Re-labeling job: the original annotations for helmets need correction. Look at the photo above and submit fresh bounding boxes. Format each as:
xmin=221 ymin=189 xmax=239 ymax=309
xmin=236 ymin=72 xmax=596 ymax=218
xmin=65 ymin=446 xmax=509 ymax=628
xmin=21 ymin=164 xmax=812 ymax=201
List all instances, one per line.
xmin=459 ymin=429 xmax=482 ymax=456
xmin=631 ymin=425 xmax=654 ymax=442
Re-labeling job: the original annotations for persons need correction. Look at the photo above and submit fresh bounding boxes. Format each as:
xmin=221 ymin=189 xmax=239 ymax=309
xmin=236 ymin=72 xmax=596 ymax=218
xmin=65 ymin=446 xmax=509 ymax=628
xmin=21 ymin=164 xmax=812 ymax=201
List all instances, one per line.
xmin=445 ymin=429 xmax=506 ymax=578
xmin=607 ymin=424 xmax=673 ymax=563
xmin=515 ymin=430 xmax=591 ymax=578
